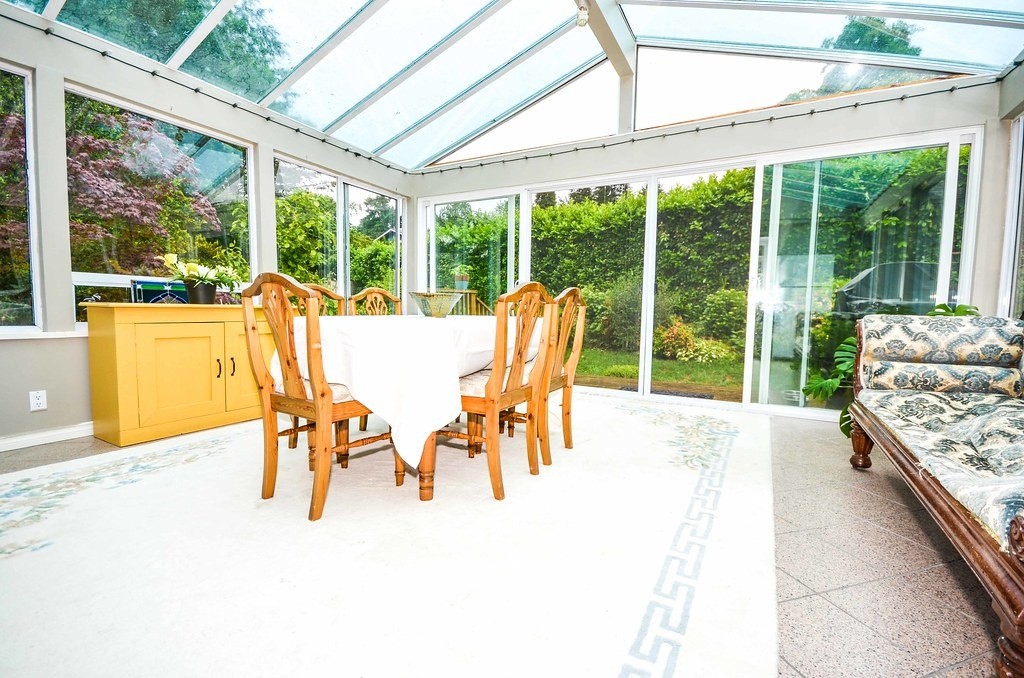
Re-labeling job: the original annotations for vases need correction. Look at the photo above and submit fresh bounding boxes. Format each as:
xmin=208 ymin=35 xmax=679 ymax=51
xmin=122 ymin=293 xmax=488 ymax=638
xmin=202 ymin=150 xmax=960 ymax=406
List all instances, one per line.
xmin=183 ymin=280 xmax=216 ymax=303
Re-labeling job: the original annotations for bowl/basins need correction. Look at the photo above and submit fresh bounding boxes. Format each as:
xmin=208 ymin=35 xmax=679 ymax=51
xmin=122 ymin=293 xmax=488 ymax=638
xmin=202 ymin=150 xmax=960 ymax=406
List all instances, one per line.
xmin=411 ymin=292 xmax=463 ymax=318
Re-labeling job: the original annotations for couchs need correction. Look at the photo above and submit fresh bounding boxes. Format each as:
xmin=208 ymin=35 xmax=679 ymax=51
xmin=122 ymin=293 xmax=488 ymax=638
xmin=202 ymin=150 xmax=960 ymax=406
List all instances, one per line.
xmin=851 ymin=313 xmax=1024 ymax=678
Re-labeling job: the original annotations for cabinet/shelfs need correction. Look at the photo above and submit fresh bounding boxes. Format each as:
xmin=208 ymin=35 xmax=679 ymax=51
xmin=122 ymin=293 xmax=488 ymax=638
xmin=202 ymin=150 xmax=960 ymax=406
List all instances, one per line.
xmin=78 ymin=301 xmax=305 ymax=448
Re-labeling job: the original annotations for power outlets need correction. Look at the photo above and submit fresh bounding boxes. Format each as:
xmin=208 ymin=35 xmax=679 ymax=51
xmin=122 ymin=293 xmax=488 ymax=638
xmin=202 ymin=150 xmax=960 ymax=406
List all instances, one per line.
xmin=29 ymin=390 xmax=47 ymax=412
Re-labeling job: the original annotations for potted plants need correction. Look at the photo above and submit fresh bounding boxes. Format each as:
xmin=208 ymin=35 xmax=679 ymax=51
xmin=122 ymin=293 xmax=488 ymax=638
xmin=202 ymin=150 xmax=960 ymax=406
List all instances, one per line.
xmin=449 ymin=265 xmax=473 ymax=289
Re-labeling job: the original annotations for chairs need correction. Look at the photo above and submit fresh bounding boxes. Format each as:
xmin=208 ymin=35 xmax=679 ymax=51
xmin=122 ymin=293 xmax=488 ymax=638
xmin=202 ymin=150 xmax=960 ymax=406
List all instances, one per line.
xmin=274 ymin=283 xmax=358 ymax=463
xmin=349 ymin=287 xmax=401 ymax=431
xmin=393 ymin=281 xmax=556 ymax=500
xmin=241 ymin=271 xmax=405 ymax=522
xmin=465 ymin=287 xmax=587 ymax=465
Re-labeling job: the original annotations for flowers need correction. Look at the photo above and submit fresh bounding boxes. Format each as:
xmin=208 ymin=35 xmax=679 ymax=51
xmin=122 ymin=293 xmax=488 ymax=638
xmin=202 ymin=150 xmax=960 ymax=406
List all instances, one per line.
xmin=154 ymin=254 xmax=242 ymax=294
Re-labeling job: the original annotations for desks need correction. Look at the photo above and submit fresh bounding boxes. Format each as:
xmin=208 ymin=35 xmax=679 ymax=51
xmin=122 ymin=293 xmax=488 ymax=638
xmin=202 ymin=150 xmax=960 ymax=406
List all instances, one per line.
xmin=285 ymin=315 xmax=549 ymax=501
